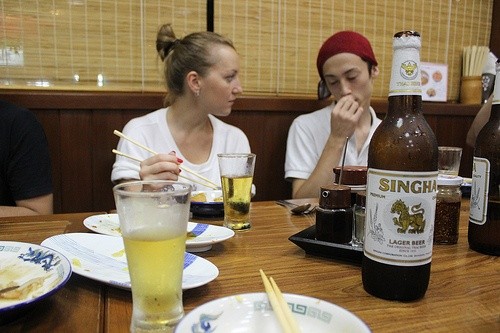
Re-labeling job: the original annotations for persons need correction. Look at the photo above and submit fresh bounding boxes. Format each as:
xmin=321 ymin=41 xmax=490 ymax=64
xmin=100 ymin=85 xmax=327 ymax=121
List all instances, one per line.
xmin=110 ymin=22 xmax=257 ymax=204
xmin=467 ymin=91 xmax=493 ymax=149
xmin=284 ymin=31 xmax=384 ymax=200
xmin=0 ymin=100 xmax=67 ymax=217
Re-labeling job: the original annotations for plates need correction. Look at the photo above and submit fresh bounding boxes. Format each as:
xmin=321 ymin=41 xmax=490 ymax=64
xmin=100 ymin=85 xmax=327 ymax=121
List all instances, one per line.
xmin=0 ymin=240 xmax=72 ymax=313
xmin=175 ymin=293 xmax=373 ymax=333
xmin=82 ymin=213 xmax=234 ymax=252
xmin=42 ymin=232 xmax=219 ymax=289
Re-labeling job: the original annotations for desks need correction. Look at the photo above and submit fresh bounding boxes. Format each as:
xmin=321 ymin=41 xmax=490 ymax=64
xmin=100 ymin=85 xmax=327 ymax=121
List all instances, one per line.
xmin=0 ymin=196 xmax=500 ymax=333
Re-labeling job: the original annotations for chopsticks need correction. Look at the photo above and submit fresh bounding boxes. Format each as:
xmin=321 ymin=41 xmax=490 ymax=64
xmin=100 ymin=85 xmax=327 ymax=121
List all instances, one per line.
xmin=462 ymin=46 xmax=490 ymax=77
xmin=259 ymin=268 xmax=302 ymax=332
xmin=112 ymin=130 xmax=221 ymax=191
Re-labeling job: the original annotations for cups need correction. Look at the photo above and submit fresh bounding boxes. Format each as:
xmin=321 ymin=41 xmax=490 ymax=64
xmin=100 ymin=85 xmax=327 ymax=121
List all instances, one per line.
xmin=114 ymin=180 xmax=193 ymax=333
xmin=218 ymin=153 xmax=257 ymax=233
xmin=438 ymin=146 xmax=463 ymax=178
xmin=460 ymin=76 xmax=481 ymax=104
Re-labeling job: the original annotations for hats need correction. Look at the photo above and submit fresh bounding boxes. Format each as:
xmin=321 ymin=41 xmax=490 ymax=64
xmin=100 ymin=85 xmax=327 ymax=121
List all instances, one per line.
xmin=317 ymin=31 xmax=377 ymax=101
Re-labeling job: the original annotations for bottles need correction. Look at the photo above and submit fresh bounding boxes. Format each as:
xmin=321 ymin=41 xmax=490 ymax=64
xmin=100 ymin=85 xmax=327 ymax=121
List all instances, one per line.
xmin=467 ymin=59 xmax=500 ymax=257
xmin=433 ymin=176 xmax=464 ymax=246
xmin=362 ymin=31 xmax=438 ymax=302
xmin=317 ymin=165 xmax=366 ymax=246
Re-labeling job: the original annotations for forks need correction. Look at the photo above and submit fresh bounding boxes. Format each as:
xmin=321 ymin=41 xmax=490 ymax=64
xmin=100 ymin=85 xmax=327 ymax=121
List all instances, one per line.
xmin=285 ymin=200 xmax=317 ymax=214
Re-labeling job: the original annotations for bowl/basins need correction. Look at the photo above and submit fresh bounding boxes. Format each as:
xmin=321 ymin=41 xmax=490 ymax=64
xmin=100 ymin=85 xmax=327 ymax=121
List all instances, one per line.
xmin=288 ymin=225 xmax=363 ymax=262
xmin=189 ymin=190 xmax=224 ymax=217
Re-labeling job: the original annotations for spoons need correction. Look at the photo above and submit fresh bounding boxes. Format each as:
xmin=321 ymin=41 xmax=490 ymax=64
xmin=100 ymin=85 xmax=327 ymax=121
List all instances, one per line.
xmin=277 ymin=200 xmax=311 ymax=213
